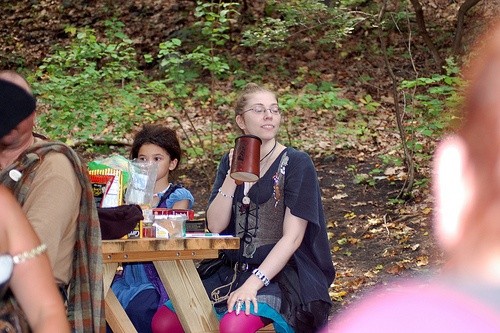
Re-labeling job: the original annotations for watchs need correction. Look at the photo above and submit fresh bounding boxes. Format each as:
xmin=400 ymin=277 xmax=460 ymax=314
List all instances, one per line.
xmin=1 ymin=245 xmax=49 ymax=284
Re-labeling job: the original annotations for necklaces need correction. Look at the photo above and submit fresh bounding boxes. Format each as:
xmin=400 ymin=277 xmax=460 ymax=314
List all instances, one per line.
xmin=260 ymin=140 xmax=276 ymax=162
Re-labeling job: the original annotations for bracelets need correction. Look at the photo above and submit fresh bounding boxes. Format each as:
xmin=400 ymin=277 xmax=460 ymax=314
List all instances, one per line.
xmin=217 ymin=189 xmax=235 ymax=199
xmin=251 ymin=268 xmax=270 ymax=286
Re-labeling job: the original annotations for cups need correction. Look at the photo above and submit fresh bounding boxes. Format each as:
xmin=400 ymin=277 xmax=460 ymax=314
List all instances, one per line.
xmin=230 ymin=134 xmax=262 ymax=182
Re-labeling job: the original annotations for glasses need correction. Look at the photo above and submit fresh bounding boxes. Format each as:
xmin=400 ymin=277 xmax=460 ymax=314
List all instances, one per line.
xmin=238 ymin=104 xmax=280 ymax=115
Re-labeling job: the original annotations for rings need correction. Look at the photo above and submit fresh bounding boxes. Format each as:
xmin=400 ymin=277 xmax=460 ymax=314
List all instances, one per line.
xmin=237 ymin=299 xmax=243 ymax=302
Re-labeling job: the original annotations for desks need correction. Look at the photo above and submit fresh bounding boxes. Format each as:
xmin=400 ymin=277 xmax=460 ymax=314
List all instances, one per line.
xmin=101 ymin=239 xmax=241 ymax=333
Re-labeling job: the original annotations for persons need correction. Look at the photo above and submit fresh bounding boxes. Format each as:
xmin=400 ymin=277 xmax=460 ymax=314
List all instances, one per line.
xmin=313 ymin=13 xmax=500 ymax=333
xmin=0 ymin=185 xmax=70 ymax=333
xmin=150 ymin=84 xmax=335 ymax=333
xmin=107 ymin=123 xmax=194 ymax=333
xmin=0 ymin=70 xmax=103 ymax=333
